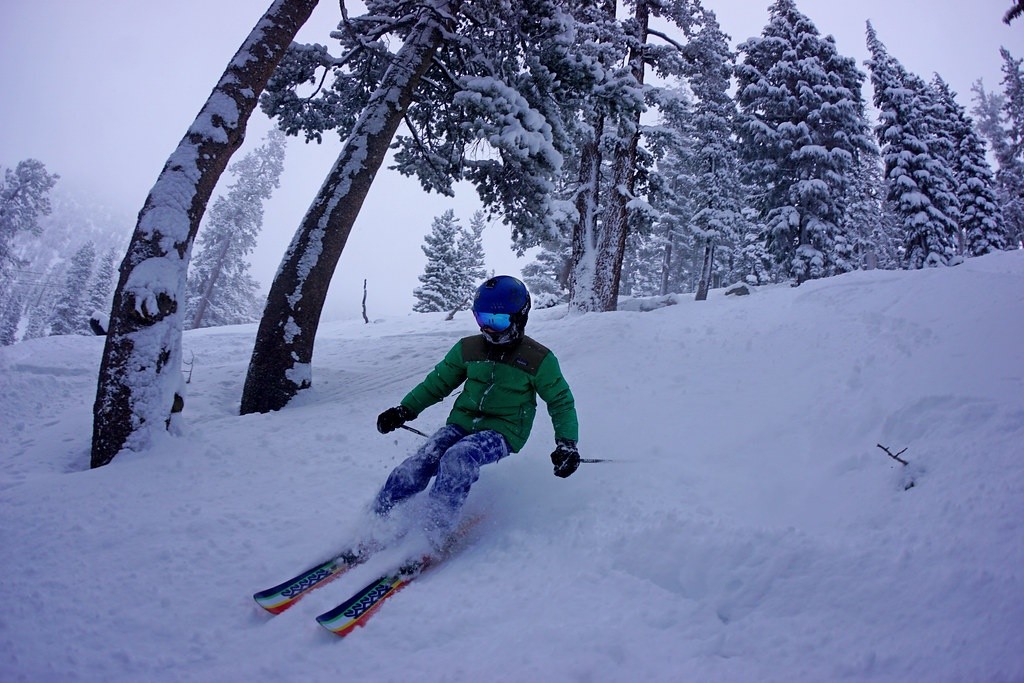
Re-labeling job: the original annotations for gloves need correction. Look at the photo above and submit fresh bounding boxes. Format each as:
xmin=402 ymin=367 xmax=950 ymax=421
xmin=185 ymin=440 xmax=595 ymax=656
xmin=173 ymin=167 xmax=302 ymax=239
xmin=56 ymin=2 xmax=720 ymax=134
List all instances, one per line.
xmin=377 ymin=405 xmax=410 ymax=434
xmin=550 ymin=440 xmax=581 ymax=478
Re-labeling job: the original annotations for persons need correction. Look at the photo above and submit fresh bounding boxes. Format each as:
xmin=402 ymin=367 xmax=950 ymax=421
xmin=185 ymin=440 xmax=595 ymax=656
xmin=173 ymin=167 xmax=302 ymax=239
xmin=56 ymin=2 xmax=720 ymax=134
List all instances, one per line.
xmin=369 ymin=275 xmax=581 ymax=575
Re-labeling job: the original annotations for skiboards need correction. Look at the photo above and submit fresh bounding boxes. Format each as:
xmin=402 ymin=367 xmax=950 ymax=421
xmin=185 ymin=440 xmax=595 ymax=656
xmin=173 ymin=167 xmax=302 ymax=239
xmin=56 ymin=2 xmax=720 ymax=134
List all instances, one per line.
xmin=253 ymin=512 xmax=487 ymax=638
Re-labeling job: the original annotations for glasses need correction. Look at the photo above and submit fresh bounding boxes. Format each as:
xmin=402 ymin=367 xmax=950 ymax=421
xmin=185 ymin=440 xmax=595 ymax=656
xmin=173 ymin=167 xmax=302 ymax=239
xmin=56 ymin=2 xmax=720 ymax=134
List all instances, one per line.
xmin=475 ymin=312 xmax=513 ymax=331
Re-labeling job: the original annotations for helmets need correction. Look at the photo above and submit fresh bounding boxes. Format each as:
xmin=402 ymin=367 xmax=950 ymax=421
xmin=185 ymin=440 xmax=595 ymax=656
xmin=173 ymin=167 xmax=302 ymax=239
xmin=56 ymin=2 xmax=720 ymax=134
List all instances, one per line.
xmin=474 ymin=276 xmax=527 ymax=344
